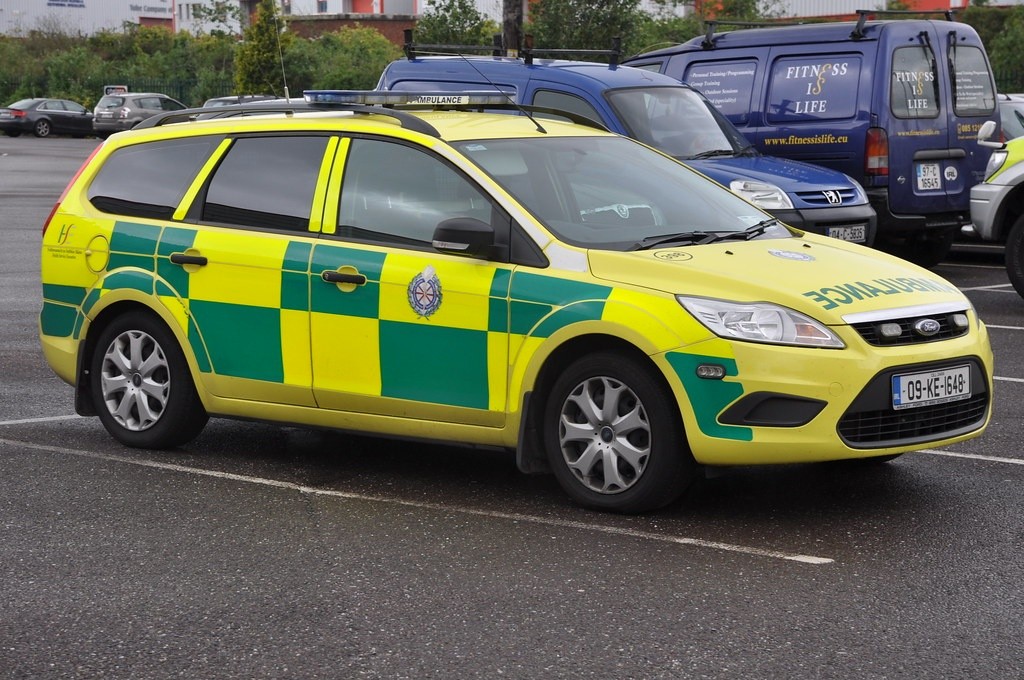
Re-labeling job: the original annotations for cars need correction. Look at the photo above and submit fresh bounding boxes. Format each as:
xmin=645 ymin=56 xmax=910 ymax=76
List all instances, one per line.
xmin=199 ymin=95 xmax=286 ymax=105
xmin=92 ymin=91 xmax=188 ymax=139
xmin=996 ymin=91 xmax=1024 ymax=142
xmin=0 ymin=98 xmax=93 ymax=138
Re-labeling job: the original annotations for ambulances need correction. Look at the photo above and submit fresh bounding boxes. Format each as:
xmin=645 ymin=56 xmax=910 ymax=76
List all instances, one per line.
xmin=36 ymin=91 xmax=993 ymax=515
xmin=959 ymin=119 xmax=1024 ymax=306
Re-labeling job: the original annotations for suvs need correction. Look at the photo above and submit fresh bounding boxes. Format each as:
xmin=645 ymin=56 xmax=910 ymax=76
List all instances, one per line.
xmin=374 ymin=23 xmax=878 ymax=245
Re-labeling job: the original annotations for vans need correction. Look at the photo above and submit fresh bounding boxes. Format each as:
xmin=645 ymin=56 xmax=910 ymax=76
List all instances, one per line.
xmin=617 ymin=8 xmax=1002 ymax=255
